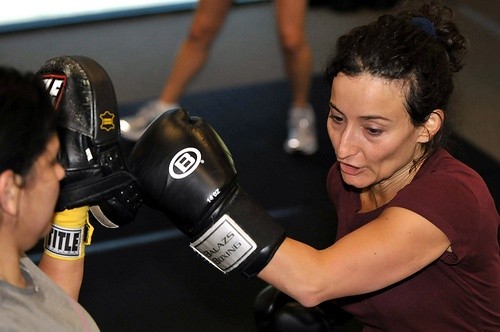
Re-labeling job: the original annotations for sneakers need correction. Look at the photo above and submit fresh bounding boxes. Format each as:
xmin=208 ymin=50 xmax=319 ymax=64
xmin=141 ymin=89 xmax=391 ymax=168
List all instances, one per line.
xmin=282 ymin=102 xmax=320 ymax=159
xmin=119 ymin=99 xmax=181 ymax=143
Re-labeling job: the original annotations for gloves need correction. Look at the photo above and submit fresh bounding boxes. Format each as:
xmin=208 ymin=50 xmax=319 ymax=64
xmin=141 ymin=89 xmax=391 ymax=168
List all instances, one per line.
xmin=124 ymin=105 xmax=291 ymax=292
xmin=253 ymin=285 xmax=335 ymax=332
xmin=35 ymin=53 xmax=135 ymax=212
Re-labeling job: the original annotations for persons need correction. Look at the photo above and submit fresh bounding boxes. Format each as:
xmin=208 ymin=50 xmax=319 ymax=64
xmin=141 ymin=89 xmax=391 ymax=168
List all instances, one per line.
xmin=0 ymin=55 xmax=121 ymax=332
xmin=124 ymin=3 xmax=500 ymax=332
xmin=119 ymin=0 xmax=318 ymax=155
